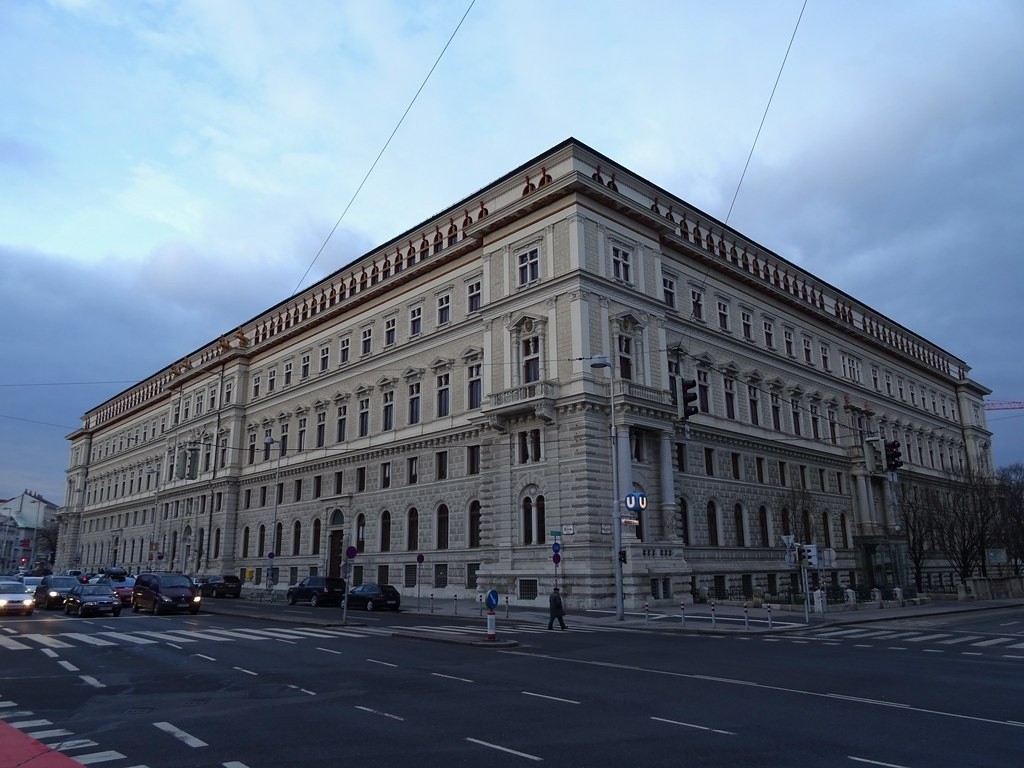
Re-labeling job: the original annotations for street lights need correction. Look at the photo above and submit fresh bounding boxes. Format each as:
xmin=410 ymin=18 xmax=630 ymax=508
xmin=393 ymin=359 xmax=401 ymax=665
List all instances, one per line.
xmin=589 ymin=353 xmax=626 ymax=622
xmin=264 ymin=436 xmax=284 ymax=603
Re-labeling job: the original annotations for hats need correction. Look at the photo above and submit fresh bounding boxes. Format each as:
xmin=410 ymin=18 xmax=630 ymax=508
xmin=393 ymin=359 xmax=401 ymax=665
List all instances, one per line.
xmin=554 ymin=588 xmax=559 ymax=591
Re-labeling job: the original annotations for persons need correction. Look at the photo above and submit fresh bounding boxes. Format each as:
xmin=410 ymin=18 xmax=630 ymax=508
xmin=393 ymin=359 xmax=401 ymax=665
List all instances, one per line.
xmin=548 ymin=588 xmax=568 ymax=630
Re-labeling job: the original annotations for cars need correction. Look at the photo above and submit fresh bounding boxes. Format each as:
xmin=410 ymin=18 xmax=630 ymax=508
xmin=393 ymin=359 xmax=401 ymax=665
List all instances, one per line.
xmin=19 ymin=576 xmax=43 ymax=597
xmin=36 ymin=575 xmax=80 ymax=610
xmin=198 ymin=575 xmax=243 ymax=597
xmin=131 ymin=573 xmax=201 ymax=614
xmin=97 ymin=567 xmax=136 ymax=608
xmin=0 ymin=581 xmax=36 ymax=616
xmin=342 ymin=583 xmax=401 ymax=611
xmin=0 ymin=575 xmax=19 ymax=581
xmin=88 ymin=573 xmax=105 ymax=584
xmin=63 ymin=583 xmax=123 ymax=617
xmin=286 ymin=575 xmax=347 ymax=608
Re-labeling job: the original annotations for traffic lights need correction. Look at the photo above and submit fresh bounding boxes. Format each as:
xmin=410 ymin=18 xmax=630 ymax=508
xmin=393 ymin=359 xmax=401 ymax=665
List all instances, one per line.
xmin=883 ymin=438 xmax=904 ymax=471
xmin=795 ymin=545 xmax=813 ymax=568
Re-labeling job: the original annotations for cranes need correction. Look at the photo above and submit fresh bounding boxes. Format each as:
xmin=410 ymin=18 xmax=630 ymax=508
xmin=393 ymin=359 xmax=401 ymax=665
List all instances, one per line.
xmin=985 ymin=400 xmax=1023 ymax=410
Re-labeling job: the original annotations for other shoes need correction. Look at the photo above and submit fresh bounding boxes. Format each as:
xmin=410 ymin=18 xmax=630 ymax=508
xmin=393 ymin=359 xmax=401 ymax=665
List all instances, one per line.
xmin=561 ymin=626 xmax=568 ymax=630
xmin=548 ymin=628 xmax=554 ymax=630
